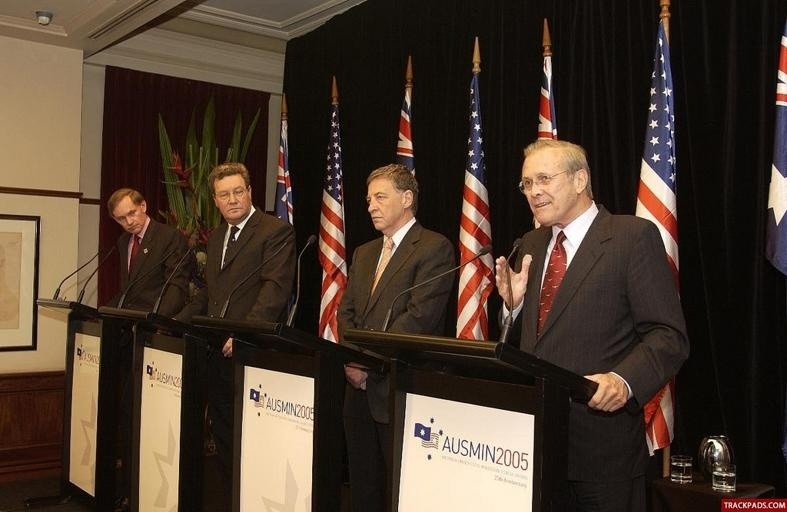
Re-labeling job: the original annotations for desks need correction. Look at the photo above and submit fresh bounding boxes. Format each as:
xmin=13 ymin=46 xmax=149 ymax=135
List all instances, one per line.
xmin=652 ymin=475 xmax=776 ymax=512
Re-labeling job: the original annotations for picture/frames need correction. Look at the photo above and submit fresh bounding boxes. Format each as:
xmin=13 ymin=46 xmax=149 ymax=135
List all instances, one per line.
xmin=0 ymin=213 xmax=41 ymax=352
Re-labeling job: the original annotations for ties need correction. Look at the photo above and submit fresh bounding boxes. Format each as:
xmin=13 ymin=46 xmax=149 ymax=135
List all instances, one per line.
xmin=370 ymin=239 xmax=395 ymax=297
xmin=129 ymin=234 xmax=141 ymax=274
xmin=223 ymin=225 xmax=240 ymax=269
xmin=537 ymin=231 xmax=566 ymax=339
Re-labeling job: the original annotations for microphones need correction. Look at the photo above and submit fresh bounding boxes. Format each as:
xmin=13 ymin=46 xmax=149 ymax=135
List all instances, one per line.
xmin=76 ymin=244 xmax=118 ymax=304
xmin=153 ymin=242 xmax=194 ymax=314
xmin=499 ymin=238 xmax=526 ymax=343
xmin=219 ymin=231 xmax=297 ymax=324
xmin=49 ymin=242 xmax=106 ymax=300
xmin=285 ymin=234 xmax=318 ymax=328
xmin=117 ymin=242 xmax=182 ymax=308
xmin=380 ymin=244 xmax=495 ymax=334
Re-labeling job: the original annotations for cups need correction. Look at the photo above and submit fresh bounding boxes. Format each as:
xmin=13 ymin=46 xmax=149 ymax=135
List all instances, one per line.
xmin=669 ymin=454 xmax=693 ymax=487
xmin=709 ymin=460 xmax=737 ymax=493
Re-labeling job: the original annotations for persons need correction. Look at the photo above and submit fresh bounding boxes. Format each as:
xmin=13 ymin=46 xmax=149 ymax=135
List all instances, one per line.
xmin=333 ymin=159 xmax=460 ymax=511
xmin=491 ymin=135 xmax=696 ymax=511
xmin=171 ymin=159 xmax=298 ymax=511
xmin=102 ymin=185 xmax=191 ymax=511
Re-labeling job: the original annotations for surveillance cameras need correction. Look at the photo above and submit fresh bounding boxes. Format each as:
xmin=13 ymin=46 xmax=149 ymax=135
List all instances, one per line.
xmin=36 ymin=11 xmax=53 ymax=26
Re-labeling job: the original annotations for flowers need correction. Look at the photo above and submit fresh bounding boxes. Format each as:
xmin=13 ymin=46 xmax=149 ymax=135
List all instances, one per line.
xmin=159 ymin=89 xmax=261 ymax=284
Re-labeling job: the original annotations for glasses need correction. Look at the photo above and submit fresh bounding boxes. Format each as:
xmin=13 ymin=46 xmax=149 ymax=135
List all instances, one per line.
xmin=218 ymin=189 xmax=246 ymax=200
xmin=519 ymin=169 xmax=568 ymax=195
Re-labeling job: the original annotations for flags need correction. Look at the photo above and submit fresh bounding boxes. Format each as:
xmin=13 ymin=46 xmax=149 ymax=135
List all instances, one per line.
xmin=760 ymin=3 xmax=787 ymax=280
xmin=272 ymin=87 xmax=301 ymax=333
xmin=631 ymin=0 xmax=682 ymax=468
xmin=529 ymin=14 xmax=560 ymax=229
xmin=392 ymin=50 xmax=419 ymax=184
xmin=451 ymin=37 xmax=494 ymax=345
xmin=313 ymin=71 xmax=354 ymax=345
